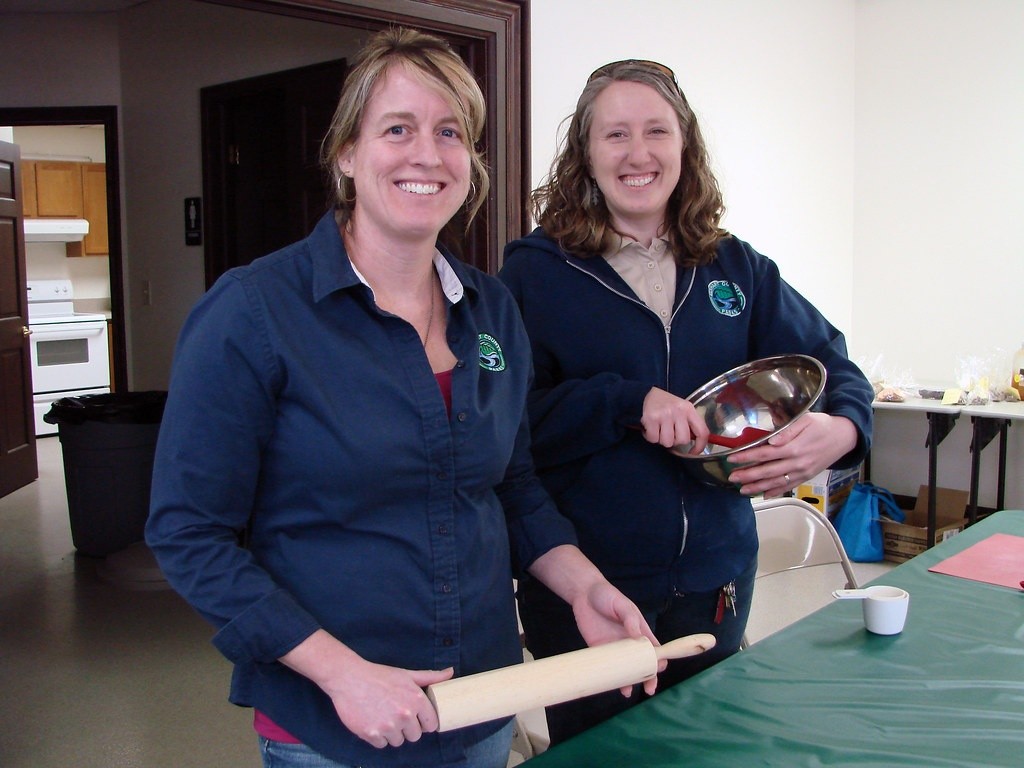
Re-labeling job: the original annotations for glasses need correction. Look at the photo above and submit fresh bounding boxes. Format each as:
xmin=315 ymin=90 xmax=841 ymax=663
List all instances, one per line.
xmin=586 ymin=60 xmax=680 ymax=84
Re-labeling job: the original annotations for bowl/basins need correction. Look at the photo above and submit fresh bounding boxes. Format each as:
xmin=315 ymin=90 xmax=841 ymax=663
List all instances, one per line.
xmin=667 ymin=354 xmax=828 ymax=481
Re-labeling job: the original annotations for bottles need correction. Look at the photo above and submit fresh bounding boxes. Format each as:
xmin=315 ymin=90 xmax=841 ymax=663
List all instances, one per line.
xmin=1011 ymin=341 xmax=1024 ymax=401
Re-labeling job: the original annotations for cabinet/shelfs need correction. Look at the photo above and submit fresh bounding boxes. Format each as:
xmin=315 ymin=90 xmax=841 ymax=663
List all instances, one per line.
xmin=19 ymin=158 xmax=110 ymax=258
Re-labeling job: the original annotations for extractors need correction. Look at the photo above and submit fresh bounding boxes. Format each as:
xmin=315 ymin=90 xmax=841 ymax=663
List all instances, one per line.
xmin=24 ymin=218 xmax=89 ymax=242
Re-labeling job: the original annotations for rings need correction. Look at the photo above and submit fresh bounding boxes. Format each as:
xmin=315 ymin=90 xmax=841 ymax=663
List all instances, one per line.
xmin=784 ymin=474 xmax=791 ymax=486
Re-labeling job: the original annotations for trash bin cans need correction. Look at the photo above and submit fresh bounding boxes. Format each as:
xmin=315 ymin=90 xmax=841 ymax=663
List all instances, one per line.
xmin=44 ymin=389 xmax=170 ymax=552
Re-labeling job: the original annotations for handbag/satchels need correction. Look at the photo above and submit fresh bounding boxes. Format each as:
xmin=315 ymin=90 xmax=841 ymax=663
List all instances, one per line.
xmin=833 ymin=480 xmax=904 ymax=563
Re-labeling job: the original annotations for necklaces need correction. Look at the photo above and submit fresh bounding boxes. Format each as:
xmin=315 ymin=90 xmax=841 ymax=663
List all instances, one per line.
xmin=423 ymin=271 xmax=435 ymax=349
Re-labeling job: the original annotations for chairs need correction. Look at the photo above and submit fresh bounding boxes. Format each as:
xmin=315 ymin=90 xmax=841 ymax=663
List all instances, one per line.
xmin=513 ymin=509 xmax=1024 ymax=768
xmin=739 ymin=497 xmax=859 ymax=650
xmin=502 ymin=646 xmax=551 ymax=761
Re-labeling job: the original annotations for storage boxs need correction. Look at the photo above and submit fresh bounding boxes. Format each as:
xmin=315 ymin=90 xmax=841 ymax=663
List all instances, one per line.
xmin=784 ymin=459 xmax=862 ymax=520
xmin=878 ymin=486 xmax=970 ymax=567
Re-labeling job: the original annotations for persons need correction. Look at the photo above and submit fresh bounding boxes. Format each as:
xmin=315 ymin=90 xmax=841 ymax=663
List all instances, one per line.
xmin=146 ymin=27 xmax=667 ymax=768
xmin=494 ymin=59 xmax=874 ymax=750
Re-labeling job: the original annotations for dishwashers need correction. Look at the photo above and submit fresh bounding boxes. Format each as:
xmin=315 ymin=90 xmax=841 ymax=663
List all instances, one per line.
xmin=29 ymin=321 xmax=111 ymax=436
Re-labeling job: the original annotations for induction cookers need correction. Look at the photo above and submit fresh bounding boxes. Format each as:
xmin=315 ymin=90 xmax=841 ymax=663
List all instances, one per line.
xmin=27 ymin=279 xmax=106 ymax=324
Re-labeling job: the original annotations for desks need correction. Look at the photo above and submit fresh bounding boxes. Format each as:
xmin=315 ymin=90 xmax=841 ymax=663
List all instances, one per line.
xmin=862 ymin=399 xmax=1024 ymax=550
